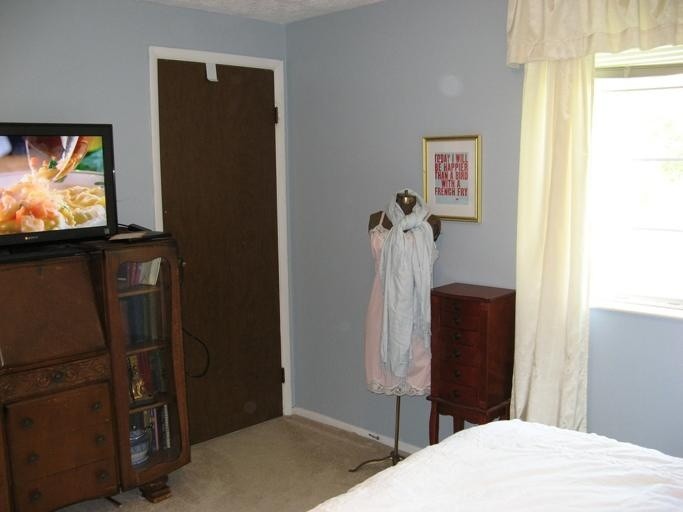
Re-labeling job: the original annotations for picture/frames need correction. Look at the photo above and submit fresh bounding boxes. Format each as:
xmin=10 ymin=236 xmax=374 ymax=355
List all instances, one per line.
xmin=420 ymin=132 xmax=481 ymax=224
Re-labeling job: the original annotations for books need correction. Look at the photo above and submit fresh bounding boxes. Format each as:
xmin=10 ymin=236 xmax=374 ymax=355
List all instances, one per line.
xmin=113 ymin=258 xmax=162 ymax=291
xmin=125 ymin=348 xmax=172 ymax=456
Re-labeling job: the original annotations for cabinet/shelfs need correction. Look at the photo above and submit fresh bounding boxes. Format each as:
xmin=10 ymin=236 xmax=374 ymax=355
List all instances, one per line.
xmin=427 ymin=281 xmax=515 ymax=445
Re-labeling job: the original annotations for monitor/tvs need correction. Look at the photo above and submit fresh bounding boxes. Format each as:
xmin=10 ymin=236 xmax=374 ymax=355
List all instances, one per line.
xmin=0 ymin=122 xmax=118 ymax=263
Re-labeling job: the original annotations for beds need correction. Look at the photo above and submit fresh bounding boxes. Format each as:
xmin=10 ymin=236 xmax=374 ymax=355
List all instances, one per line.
xmin=302 ymin=419 xmax=681 ymax=512
